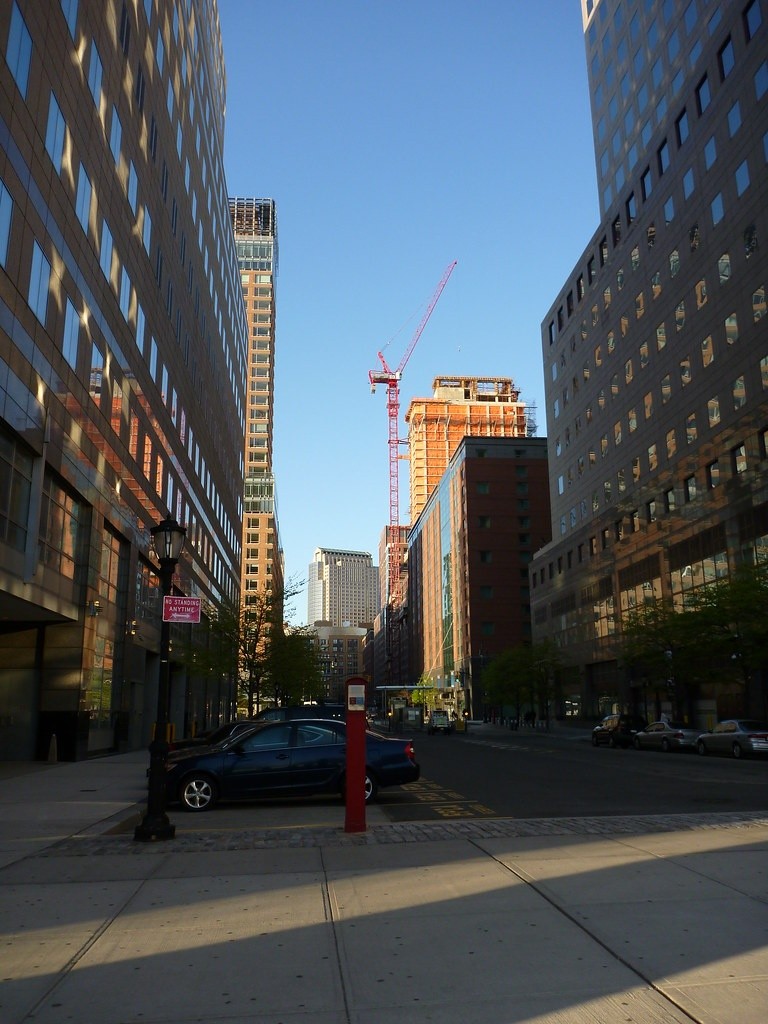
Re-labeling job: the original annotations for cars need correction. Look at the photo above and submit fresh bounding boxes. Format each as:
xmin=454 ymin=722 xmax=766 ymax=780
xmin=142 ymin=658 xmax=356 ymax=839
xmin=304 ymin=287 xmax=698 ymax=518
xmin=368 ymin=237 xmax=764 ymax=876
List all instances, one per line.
xmin=172 ymin=702 xmax=371 ymax=751
xmin=697 ymin=718 xmax=768 ymax=759
xmin=633 ymin=721 xmax=704 ymax=753
xmin=427 ymin=715 xmax=456 ymax=737
xmin=167 ymin=719 xmax=420 ymax=811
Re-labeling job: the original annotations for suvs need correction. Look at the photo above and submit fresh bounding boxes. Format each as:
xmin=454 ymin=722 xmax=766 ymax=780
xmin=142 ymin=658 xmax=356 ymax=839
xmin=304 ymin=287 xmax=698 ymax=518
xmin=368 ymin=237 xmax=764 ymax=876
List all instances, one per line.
xmin=592 ymin=714 xmax=649 ymax=749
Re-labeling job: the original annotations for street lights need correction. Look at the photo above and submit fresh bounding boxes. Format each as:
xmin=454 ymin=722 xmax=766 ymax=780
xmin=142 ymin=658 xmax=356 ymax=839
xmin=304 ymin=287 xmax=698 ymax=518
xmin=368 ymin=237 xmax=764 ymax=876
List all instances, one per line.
xmin=133 ymin=511 xmax=188 ymax=844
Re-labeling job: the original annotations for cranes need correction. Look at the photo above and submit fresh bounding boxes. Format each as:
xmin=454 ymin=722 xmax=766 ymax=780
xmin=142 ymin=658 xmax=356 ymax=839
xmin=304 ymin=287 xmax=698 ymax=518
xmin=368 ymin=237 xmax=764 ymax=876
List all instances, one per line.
xmin=369 ymin=257 xmax=458 ymax=685
